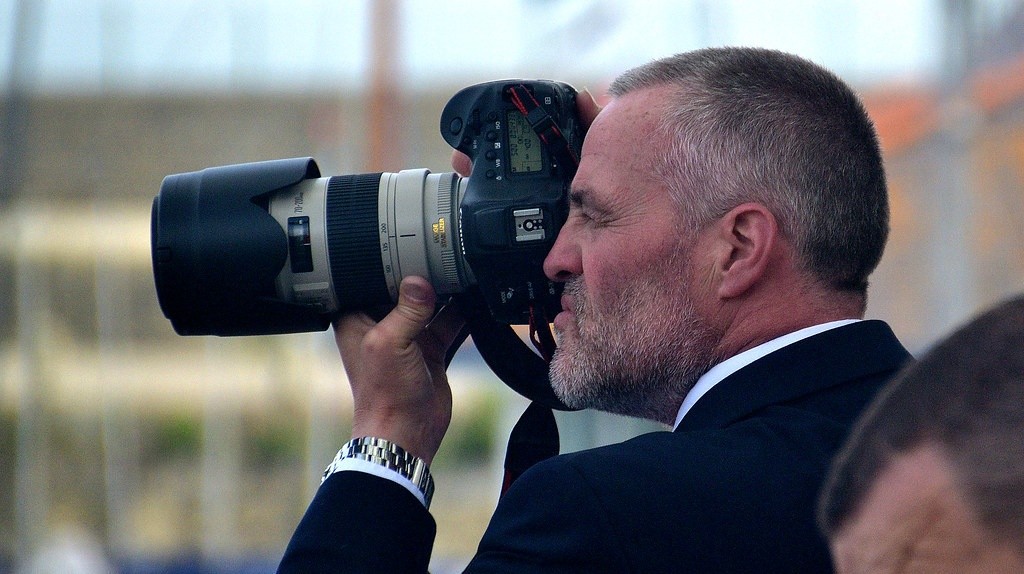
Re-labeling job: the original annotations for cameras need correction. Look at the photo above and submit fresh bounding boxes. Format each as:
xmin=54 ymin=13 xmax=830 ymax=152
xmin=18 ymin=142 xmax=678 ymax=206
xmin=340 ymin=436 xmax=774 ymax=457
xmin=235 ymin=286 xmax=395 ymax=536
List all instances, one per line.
xmin=150 ymin=79 xmax=585 ymax=337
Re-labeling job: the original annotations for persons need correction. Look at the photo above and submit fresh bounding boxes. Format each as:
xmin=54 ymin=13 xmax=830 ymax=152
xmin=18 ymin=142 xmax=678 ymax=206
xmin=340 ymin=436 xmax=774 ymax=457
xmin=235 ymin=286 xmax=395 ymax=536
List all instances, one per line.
xmin=823 ymin=296 xmax=1023 ymax=574
xmin=276 ymin=47 xmax=915 ymax=574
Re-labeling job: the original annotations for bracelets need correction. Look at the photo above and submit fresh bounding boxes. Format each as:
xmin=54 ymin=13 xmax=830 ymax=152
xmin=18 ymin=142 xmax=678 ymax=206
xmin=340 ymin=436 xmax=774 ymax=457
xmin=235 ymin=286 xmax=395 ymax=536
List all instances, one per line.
xmin=321 ymin=438 xmax=436 ymax=511
